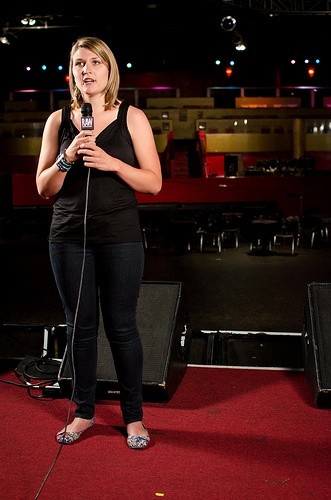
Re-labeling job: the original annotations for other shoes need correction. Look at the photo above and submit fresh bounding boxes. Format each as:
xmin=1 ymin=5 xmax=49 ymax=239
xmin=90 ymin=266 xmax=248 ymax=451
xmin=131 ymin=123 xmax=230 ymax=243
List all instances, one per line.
xmin=56 ymin=417 xmax=95 ymax=445
xmin=126 ymin=423 xmax=151 ymax=449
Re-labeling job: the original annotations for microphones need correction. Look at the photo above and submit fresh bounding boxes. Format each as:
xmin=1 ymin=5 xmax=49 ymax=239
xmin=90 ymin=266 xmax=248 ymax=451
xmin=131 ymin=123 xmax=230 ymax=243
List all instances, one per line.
xmin=81 ymin=103 xmax=94 ymax=131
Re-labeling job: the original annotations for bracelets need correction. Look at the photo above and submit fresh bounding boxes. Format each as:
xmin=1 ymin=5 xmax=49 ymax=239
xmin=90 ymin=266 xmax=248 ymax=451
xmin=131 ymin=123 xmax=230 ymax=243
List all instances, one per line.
xmin=55 ymin=150 xmax=73 ymax=172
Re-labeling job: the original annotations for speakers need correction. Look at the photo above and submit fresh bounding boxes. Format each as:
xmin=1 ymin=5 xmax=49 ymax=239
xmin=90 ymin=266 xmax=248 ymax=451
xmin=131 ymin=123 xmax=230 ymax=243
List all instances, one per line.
xmin=58 ymin=281 xmax=188 ymax=400
xmin=302 ymin=282 xmax=331 ymax=408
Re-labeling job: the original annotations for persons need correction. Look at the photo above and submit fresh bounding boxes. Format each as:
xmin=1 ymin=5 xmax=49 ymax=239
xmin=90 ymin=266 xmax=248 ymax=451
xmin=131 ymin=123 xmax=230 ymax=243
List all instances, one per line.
xmin=35 ymin=37 xmax=164 ymax=448
